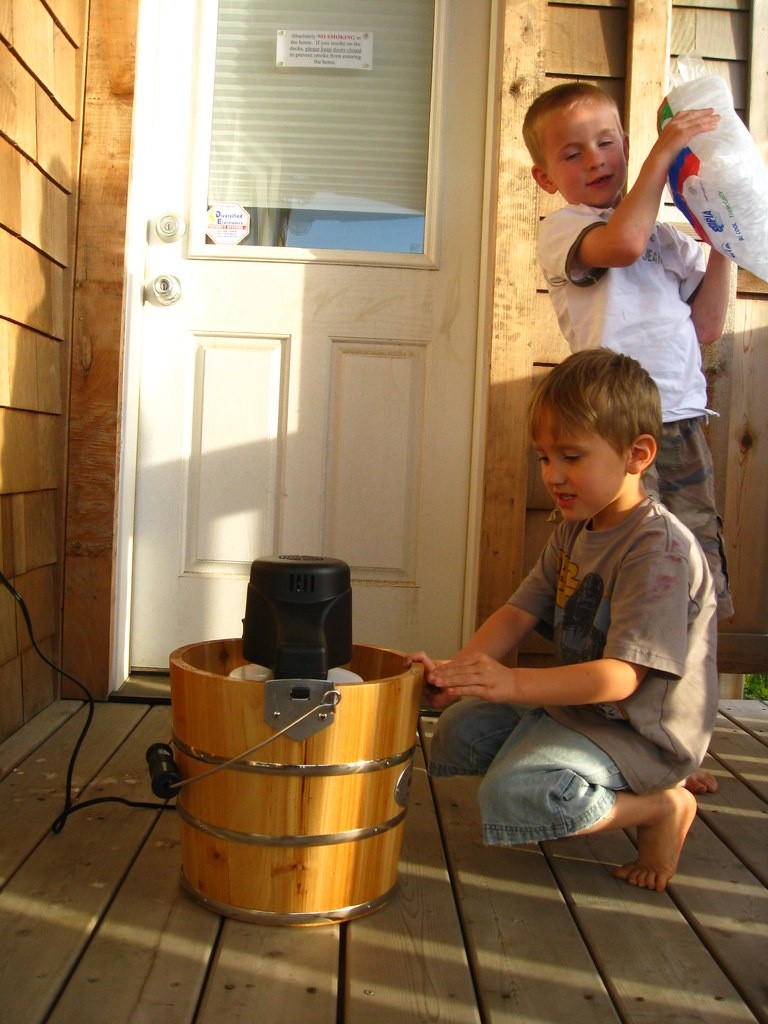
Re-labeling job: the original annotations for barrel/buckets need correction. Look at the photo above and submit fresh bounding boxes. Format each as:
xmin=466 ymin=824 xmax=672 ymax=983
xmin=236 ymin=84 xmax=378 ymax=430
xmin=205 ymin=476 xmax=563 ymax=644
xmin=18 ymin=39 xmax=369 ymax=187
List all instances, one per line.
xmin=171 ymin=637 xmax=424 ymax=927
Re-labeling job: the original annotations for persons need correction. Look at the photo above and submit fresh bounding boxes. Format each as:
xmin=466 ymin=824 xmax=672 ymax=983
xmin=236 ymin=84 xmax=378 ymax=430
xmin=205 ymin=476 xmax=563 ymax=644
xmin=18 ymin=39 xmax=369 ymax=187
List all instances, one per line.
xmin=522 ymin=83 xmax=731 ymax=796
xmin=402 ymin=346 xmax=717 ymax=895
